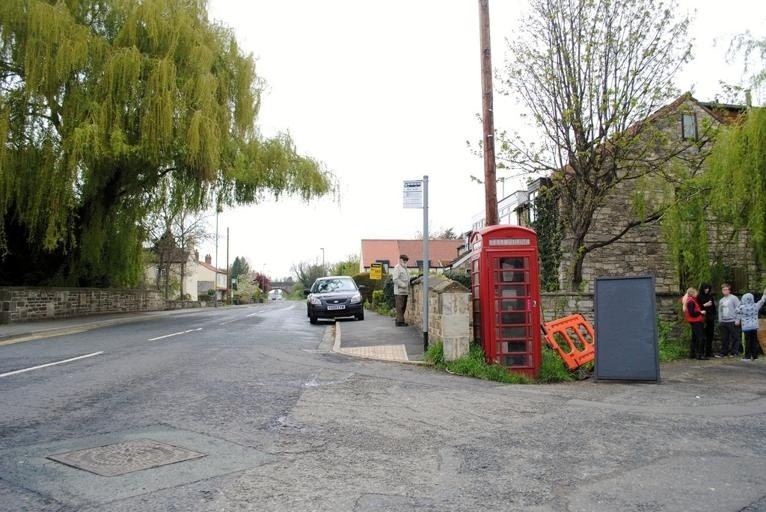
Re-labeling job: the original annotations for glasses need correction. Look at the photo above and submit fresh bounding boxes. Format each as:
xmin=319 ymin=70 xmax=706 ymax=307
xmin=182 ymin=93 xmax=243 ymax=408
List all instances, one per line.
xmin=403 ymin=258 xmax=408 ymax=261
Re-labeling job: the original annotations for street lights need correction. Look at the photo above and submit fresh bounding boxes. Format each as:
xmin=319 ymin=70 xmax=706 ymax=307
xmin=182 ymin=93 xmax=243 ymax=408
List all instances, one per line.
xmin=320 ymin=248 xmax=324 ymax=275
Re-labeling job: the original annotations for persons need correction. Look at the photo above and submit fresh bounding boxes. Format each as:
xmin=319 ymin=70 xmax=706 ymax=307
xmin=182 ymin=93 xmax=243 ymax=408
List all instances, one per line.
xmin=733 ymin=288 xmax=766 ymax=362
xmin=680 ymin=286 xmax=710 ymax=362
xmin=390 ymin=253 xmax=411 ymax=327
xmin=712 ymin=282 xmax=740 ymax=359
xmin=695 ymin=281 xmax=716 ymax=358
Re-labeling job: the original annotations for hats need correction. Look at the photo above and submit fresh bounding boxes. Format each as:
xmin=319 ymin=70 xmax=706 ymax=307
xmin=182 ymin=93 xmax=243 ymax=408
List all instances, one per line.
xmin=400 ymin=255 xmax=409 ymax=259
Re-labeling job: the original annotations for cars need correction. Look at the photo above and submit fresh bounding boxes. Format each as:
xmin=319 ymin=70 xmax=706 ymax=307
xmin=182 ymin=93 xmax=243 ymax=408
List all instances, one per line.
xmin=303 ymin=276 xmax=364 ymax=325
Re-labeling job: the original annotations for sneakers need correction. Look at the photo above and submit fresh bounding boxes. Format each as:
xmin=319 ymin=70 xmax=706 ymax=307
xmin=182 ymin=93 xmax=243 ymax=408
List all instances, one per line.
xmin=395 ymin=320 xmax=408 ymax=326
xmin=688 ymin=352 xmax=758 ymax=361
